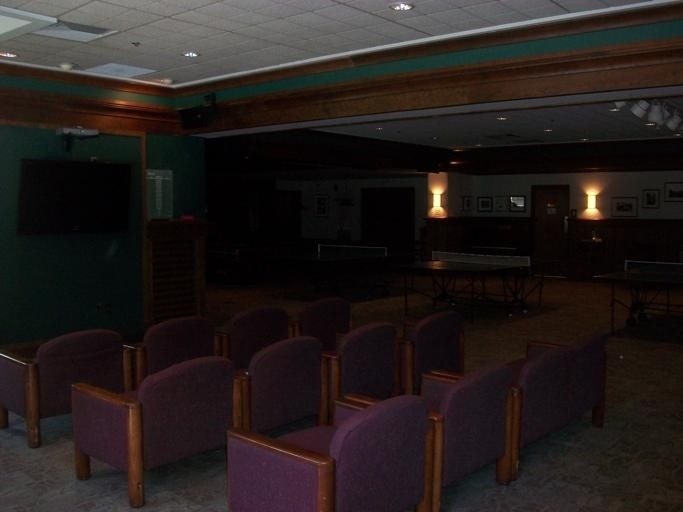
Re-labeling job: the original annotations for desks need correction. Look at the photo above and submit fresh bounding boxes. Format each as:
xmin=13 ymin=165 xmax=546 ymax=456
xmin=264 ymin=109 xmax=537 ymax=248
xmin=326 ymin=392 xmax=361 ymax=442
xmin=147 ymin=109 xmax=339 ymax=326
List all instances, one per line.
xmin=401 ymin=249 xmax=545 ymax=323
xmin=591 ymin=259 xmax=682 ymax=337
xmin=282 ymin=241 xmax=408 ymax=303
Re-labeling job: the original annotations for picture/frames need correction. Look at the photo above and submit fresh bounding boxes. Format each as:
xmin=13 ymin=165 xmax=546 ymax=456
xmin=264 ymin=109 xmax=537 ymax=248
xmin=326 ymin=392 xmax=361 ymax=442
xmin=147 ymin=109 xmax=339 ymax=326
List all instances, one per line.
xmin=608 ymin=180 xmax=683 ymax=217
xmin=313 ymin=194 xmax=329 ymax=218
xmin=459 ymin=180 xmax=526 ymax=214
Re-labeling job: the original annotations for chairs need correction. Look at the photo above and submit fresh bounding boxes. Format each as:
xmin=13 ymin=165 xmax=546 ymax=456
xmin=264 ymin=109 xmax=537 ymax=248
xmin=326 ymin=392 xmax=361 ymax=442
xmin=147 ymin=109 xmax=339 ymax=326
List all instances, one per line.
xmin=428 ymin=330 xmax=609 ymax=479
xmin=400 ymin=311 xmax=464 ymax=397
xmin=321 ymin=321 xmax=414 ymax=424
xmin=123 ymin=316 xmax=223 ymax=391
xmin=70 ymin=355 xmax=242 ymax=507
xmin=343 ymin=365 xmax=513 ymax=509
xmin=241 ymin=337 xmax=329 ymax=430
xmin=2 ymin=328 xmax=136 ymax=449
xmin=227 ymin=399 xmax=434 ymax=509
xmin=223 ymin=297 xmax=353 ymax=369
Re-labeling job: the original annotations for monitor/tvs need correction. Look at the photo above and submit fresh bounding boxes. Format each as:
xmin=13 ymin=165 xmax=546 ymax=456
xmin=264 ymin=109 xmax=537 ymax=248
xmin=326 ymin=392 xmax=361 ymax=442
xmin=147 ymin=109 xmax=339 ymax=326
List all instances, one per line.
xmin=16 ymin=159 xmax=131 ymax=235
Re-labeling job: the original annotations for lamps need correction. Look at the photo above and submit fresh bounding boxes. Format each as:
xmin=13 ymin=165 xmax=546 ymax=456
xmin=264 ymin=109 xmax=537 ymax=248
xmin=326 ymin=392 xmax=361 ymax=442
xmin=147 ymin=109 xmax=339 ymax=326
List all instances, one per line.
xmin=586 ymin=195 xmax=596 ymax=208
xmin=432 ymin=194 xmax=441 ymax=207
xmin=632 ymin=98 xmax=683 ymax=137
